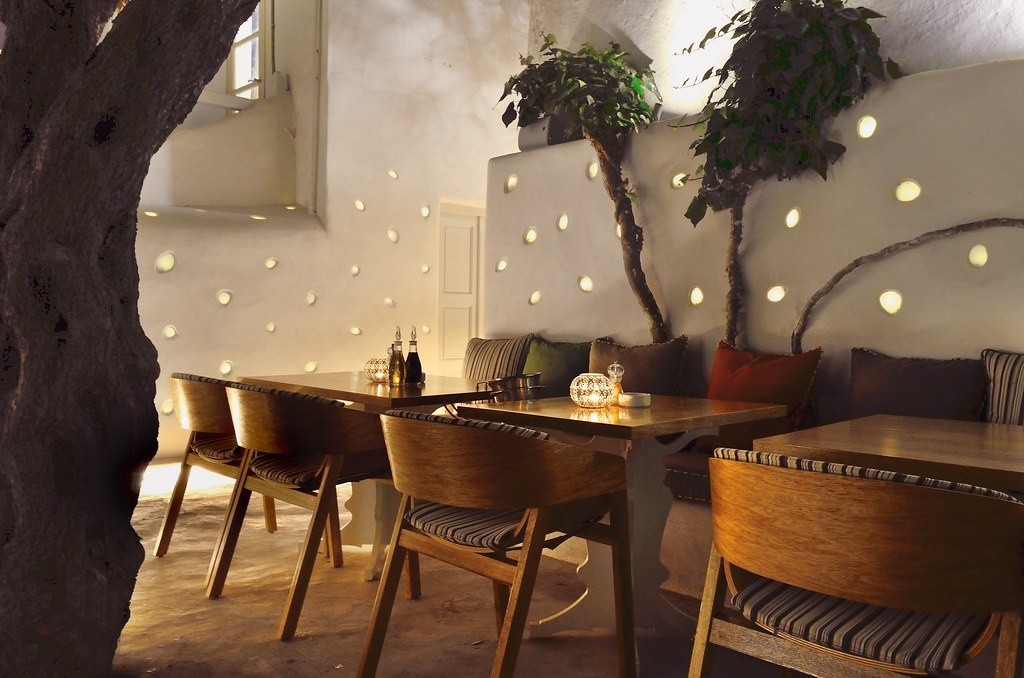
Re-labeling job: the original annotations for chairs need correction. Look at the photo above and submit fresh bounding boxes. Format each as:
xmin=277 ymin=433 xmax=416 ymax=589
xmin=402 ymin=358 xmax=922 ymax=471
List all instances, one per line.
xmin=202 ymin=382 xmax=422 ymax=640
xmin=154 ymin=372 xmax=331 ymax=565
xmin=688 ymin=446 xmax=1024 ymax=678
xmin=356 ymin=410 xmax=638 ymax=678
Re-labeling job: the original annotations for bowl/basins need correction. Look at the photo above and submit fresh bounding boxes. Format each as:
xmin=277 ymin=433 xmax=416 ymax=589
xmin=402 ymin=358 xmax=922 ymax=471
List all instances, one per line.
xmin=490 ymin=385 xmax=548 ymax=402
xmin=488 ymin=372 xmax=541 ymax=392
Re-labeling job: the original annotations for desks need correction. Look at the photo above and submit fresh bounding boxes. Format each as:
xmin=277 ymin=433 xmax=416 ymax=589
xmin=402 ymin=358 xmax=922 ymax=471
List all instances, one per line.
xmin=456 ymin=391 xmax=789 ymax=639
xmin=753 ymin=413 xmax=1024 ymax=494
xmin=234 ymin=368 xmax=494 ymax=583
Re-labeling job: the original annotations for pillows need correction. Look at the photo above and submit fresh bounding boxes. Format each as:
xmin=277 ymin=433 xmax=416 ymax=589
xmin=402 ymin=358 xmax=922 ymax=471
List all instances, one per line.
xmin=588 ymin=334 xmax=688 ymax=396
xmin=694 ymin=339 xmax=823 ymax=454
xmin=842 ymin=347 xmax=990 ymax=422
xmin=522 ymin=335 xmax=618 ymax=400
xmin=981 ymin=349 xmax=1024 ymax=425
xmin=461 ymin=333 xmax=534 ymax=404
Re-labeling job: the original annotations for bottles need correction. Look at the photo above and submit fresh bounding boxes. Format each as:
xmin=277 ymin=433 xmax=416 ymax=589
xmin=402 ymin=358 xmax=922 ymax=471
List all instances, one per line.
xmin=406 ymin=341 xmax=421 ymax=385
xmin=389 ymin=341 xmax=406 ymax=385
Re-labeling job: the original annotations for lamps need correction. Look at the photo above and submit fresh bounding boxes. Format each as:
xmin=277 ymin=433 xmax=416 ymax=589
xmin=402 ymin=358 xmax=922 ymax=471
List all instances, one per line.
xmin=570 ymin=373 xmax=621 ymax=408
xmin=364 ymin=358 xmax=390 ymax=383
